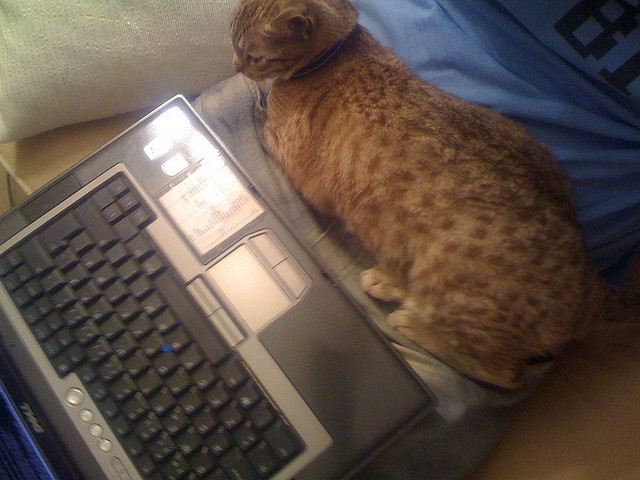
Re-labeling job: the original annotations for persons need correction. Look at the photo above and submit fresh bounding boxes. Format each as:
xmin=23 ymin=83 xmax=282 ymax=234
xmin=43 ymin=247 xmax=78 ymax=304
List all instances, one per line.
xmin=189 ymin=0 xmax=636 ymax=478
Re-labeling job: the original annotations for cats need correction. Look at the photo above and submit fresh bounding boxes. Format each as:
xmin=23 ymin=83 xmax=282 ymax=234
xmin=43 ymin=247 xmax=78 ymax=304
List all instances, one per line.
xmin=230 ymin=1 xmax=639 ymax=389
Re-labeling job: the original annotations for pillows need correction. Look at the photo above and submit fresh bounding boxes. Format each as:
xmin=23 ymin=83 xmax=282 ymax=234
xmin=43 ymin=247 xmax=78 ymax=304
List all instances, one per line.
xmin=1 ymin=0 xmax=241 ymax=143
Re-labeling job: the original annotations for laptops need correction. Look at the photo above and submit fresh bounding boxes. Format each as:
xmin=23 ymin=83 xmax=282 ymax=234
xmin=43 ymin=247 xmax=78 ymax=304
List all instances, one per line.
xmin=0 ymin=93 xmax=438 ymax=480
